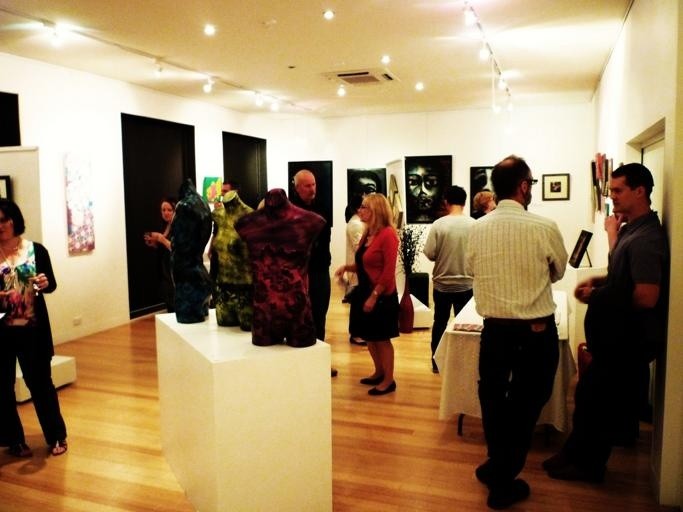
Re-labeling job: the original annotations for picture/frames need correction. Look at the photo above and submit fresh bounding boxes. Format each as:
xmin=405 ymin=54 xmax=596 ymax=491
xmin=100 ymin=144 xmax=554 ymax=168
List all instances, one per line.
xmin=568 ymin=229 xmax=593 ymax=267
xmin=0 ymin=174 xmax=14 ymax=202
xmin=541 ymin=172 xmax=570 ymax=202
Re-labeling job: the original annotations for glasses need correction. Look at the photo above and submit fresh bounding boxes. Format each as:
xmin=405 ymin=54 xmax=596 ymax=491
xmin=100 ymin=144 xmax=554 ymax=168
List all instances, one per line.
xmin=530 ymin=180 xmax=537 ymax=185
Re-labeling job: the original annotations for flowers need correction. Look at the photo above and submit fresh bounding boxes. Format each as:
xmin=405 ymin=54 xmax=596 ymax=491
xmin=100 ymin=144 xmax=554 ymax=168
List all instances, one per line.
xmin=395 ymin=223 xmax=428 ymax=281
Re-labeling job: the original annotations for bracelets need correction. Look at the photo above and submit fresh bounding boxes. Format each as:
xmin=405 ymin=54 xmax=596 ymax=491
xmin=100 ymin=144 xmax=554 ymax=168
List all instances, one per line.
xmin=372 ymin=289 xmax=379 ymax=297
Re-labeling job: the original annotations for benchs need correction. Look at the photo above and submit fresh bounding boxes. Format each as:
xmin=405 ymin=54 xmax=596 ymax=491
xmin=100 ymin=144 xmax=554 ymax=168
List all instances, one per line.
xmin=399 ymin=292 xmax=434 ymax=330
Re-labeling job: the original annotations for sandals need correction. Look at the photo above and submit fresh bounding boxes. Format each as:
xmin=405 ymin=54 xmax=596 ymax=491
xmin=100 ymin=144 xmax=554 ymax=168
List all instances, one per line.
xmin=49 ymin=438 xmax=67 ymax=455
xmin=8 ymin=442 xmax=33 ymax=457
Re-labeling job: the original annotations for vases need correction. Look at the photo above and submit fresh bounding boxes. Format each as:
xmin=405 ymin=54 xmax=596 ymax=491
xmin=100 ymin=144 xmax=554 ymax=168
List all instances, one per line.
xmin=398 ymin=281 xmax=415 ymax=335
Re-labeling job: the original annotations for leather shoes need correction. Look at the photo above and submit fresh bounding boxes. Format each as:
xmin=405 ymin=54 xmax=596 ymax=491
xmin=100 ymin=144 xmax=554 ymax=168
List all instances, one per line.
xmin=550 ymin=464 xmax=581 ymax=479
xmin=542 ymin=456 xmax=564 ymax=469
xmin=487 ymin=479 xmax=530 ymax=511
xmin=360 ymin=374 xmax=384 ymax=385
xmin=368 ymin=380 xmax=396 ymax=395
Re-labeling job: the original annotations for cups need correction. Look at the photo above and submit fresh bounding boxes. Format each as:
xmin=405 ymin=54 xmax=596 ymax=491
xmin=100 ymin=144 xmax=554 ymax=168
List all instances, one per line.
xmin=144 ymin=233 xmax=149 ymax=246
xmin=27 ymin=277 xmax=37 ymax=296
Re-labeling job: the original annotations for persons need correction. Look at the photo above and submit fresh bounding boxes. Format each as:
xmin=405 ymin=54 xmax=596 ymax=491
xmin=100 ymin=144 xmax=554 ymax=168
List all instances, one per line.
xmin=406 ymin=163 xmax=439 ymax=224
xmin=348 ymin=171 xmax=380 ymax=203
xmin=169 ymin=185 xmax=212 ymax=324
xmin=463 ymin=154 xmax=569 ymax=509
xmin=0 ymin=197 xmax=67 ymax=458
xmin=472 ymin=192 xmax=496 ymax=219
xmin=208 ymin=180 xmax=242 ymax=309
xmin=142 ymin=199 xmax=176 ymax=313
xmin=542 ymin=162 xmax=668 ymax=485
xmin=289 ymin=169 xmax=338 ymax=377
xmin=211 ymin=190 xmax=256 ymax=330
xmin=345 ymin=198 xmax=369 ymax=346
xmin=334 ymin=194 xmax=400 ymax=396
xmin=232 ymin=188 xmax=326 ymax=348
xmin=422 ymin=186 xmax=477 ymax=374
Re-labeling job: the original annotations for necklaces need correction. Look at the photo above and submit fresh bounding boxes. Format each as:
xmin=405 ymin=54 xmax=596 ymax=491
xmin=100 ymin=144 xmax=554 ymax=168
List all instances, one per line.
xmin=2 ymin=241 xmax=18 ymax=253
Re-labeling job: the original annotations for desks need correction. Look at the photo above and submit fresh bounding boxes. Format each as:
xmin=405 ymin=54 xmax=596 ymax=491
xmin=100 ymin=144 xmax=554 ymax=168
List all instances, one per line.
xmin=446 ymin=289 xmax=571 ymax=436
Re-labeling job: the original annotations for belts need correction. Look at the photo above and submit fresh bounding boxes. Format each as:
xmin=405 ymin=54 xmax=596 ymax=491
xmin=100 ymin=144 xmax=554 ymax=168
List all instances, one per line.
xmin=484 ymin=313 xmax=555 ymax=326
